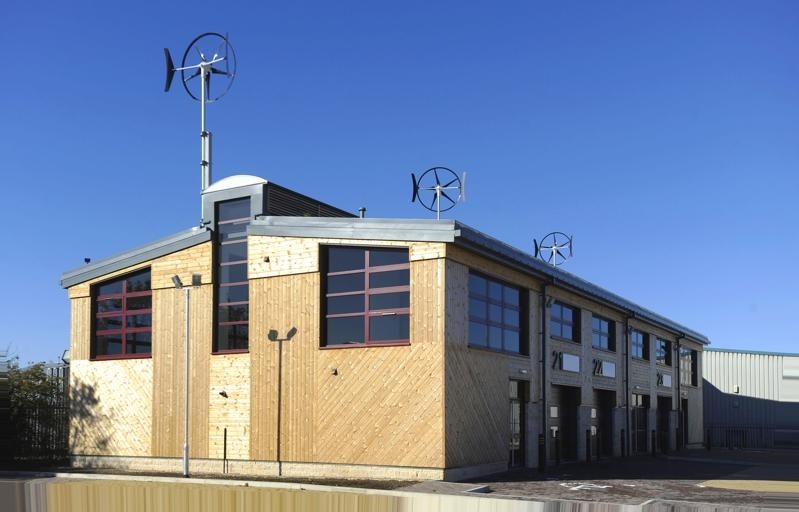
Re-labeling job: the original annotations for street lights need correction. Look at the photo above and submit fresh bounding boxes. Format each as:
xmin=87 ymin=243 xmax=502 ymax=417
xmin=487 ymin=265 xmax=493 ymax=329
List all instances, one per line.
xmin=166 ymin=270 xmax=210 ymax=477
xmin=265 ymin=322 xmax=302 ymax=477
